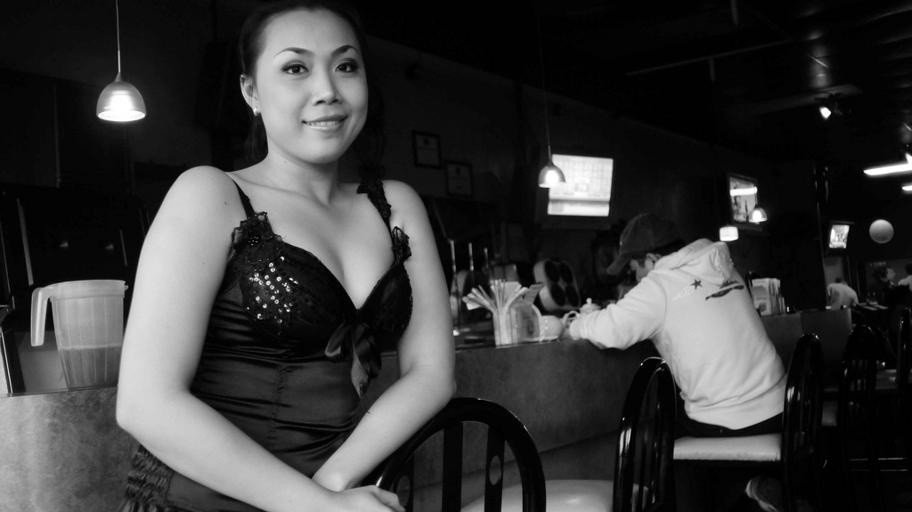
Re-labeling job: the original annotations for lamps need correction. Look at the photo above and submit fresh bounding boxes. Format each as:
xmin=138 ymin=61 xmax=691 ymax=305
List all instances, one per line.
xmin=95 ymin=1 xmax=147 ymax=123
xmin=537 ymin=39 xmax=567 ymax=189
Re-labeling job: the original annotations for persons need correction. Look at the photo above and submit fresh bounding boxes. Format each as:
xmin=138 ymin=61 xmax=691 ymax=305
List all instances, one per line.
xmin=567 ymin=212 xmax=801 ymax=512
xmin=825 ymin=276 xmax=860 ymax=311
xmin=898 ymin=262 xmax=912 ymax=291
xmin=113 ymin=0 xmax=458 ymax=512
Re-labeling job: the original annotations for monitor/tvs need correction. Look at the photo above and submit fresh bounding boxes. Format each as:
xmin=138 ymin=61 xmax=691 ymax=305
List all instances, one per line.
xmin=727 ymin=172 xmax=765 ymax=228
xmin=827 ymin=219 xmax=854 ymax=257
xmin=537 ymin=154 xmax=620 ymax=224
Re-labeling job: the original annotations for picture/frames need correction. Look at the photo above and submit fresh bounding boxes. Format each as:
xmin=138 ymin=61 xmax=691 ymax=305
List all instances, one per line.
xmin=411 ymin=129 xmax=442 ymax=170
xmin=444 ymin=159 xmax=475 ymax=199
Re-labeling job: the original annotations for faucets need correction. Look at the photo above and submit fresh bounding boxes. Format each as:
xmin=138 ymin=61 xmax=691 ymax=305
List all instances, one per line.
xmin=446 ymin=237 xmax=461 ymax=326
xmin=482 ymin=247 xmax=490 ymax=286
xmin=469 ymin=242 xmax=475 ymax=288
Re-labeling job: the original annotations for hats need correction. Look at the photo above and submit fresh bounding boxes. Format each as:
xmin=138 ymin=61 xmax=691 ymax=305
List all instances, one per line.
xmin=607 ymin=213 xmax=677 ymax=276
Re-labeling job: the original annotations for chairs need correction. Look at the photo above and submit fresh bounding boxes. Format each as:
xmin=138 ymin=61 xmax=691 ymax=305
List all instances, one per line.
xmin=368 ymin=398 xmax=547 ymax=512
xmin=612 ymin=316 xmax=911 ymax=512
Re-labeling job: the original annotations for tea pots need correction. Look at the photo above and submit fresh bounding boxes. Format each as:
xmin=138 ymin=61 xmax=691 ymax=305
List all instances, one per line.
xmin=539 ymin=312 xmax=571 ymax=340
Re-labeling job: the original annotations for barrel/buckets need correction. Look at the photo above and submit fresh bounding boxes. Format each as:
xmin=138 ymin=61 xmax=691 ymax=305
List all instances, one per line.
xmin=27 ymin=280 xmax=126 ymax=389
